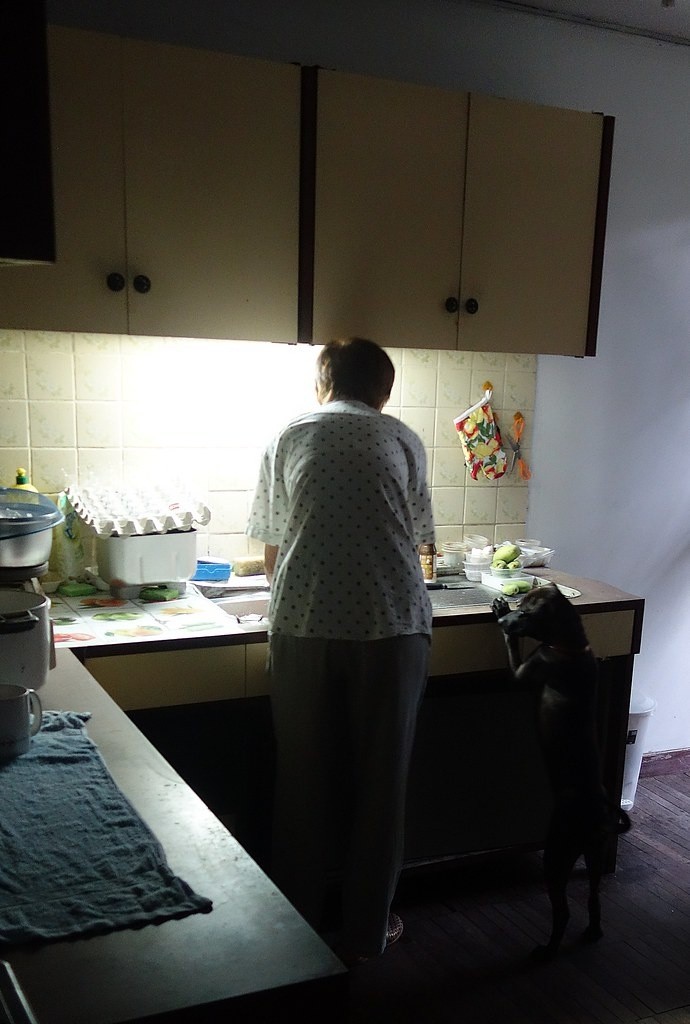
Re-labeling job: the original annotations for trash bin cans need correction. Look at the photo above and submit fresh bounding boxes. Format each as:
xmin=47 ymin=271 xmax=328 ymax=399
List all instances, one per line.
xmin=620 ymin=693 xmax=658 ymax=811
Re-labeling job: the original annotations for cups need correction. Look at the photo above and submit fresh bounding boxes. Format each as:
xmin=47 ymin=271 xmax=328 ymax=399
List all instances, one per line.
xmin=0 ymin=684 xmax=42 ymax=756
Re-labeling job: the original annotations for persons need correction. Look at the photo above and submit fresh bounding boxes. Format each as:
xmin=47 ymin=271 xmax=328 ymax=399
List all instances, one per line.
xmin=244 ymin=336 xmax=437 ymax=965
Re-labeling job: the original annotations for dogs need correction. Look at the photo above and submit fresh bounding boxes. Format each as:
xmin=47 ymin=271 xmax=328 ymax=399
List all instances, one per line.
xmin=490 ymin=581 xmax=634 ymax=964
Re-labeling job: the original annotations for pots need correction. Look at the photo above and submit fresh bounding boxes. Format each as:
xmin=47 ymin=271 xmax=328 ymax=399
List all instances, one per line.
xmin=0 ymin=583 xmax=51 ymax=691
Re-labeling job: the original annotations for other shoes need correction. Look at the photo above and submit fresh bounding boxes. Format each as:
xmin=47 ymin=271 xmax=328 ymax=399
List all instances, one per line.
xmin=343 ymin=911 xmax=404 ymax=967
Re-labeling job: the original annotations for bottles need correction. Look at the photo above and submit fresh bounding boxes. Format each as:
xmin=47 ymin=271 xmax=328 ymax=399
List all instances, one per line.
xmin=419 ymin=544 xmax=437 ymax=583
xmin=58 ymin=491 xmax=85 ymax=581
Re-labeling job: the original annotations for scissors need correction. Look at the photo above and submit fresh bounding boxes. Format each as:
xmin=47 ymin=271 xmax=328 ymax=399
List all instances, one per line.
xmin=504 ymin=419 xmax=532 ymax=481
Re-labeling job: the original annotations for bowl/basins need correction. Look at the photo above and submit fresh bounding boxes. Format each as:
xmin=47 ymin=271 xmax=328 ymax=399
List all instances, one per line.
xmin=0 ymin=503 xmax=58 ymax=567
xmin=441 ymin=534 xmax=555 ymax=595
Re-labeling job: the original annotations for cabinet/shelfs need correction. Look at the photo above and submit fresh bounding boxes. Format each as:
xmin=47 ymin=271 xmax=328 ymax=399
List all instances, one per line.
xmin=298 ymin=65 xmax=618 ymax=363
xmin=1 ymin=25 xmax=303 ymax=347
